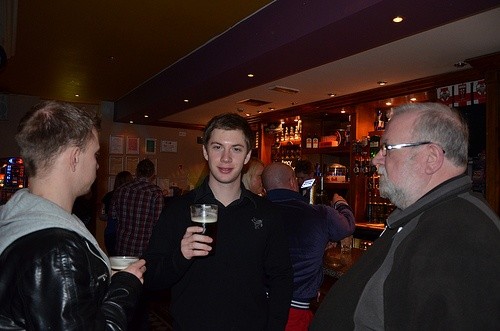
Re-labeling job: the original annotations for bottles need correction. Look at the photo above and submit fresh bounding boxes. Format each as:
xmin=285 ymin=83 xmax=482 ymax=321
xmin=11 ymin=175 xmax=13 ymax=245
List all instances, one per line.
xmin=312 ymin=135 xmax=320 ymax=148
xmin=366 ymin=173 xmax=397 ymax=222
xmin=306 ymin=135 xmax=312 ymax=149
xmin=271 ymin=125 xmax=300 ymax=161
xmin=374 ymin=107 xmax=384 ymax=129
xmin=316 ymin=162 xmax=320 ymax=176
xmin=360 ymin=240 xmax=373 ymax=250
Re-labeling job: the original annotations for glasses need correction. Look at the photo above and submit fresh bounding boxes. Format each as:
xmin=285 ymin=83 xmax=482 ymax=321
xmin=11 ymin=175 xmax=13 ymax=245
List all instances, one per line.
xmin=375 ymin=140 xmax=446 ymax=158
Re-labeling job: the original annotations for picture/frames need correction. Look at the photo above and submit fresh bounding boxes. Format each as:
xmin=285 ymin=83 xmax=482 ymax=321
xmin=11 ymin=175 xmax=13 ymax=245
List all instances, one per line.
xmin=145 ymin=138 xmax=157 ymax=155
xmin=109 ymin=134 xmax=124 ymax=155
xmin=126 ymin=135 xmax=139 ymax=155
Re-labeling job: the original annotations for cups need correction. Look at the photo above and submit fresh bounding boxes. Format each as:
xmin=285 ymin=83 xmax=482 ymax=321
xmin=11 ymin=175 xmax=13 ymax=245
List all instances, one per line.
xmin=110 ymin=256 xmax=139 ymax=276
xmin=190 ymin=203 xmax=218 ymax=255
xmin=341 ymin=235 xmax=353 ymax=252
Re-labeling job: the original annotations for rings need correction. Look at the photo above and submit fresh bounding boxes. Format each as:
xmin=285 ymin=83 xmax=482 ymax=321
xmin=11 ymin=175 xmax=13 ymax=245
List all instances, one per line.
xmin=142 ymin=266 xmax=146 ymax=272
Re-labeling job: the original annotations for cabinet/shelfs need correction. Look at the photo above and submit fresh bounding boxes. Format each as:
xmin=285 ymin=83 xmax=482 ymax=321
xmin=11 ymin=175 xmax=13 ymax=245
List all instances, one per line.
xmin=246 ymin=63 xmax=487 ymax=248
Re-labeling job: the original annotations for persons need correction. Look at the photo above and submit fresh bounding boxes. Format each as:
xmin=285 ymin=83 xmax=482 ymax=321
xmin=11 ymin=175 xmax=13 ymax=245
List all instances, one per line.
xmin=109 ymin=159 xmax=165 ymax=257
xmin=0 ymin=99 xmax=147 ymax=331
xmin=260 ymin=160 xmax=356 ymax=331
xmin=309 ymin=101 xmax=500 ymax=331
xmin=98 ymin=171 xmax=133 ymax=258
xmin=143 ymin=112 xmax=294 ymax=331
xmin=241 ymin=158 xmax=264 ymax=196
